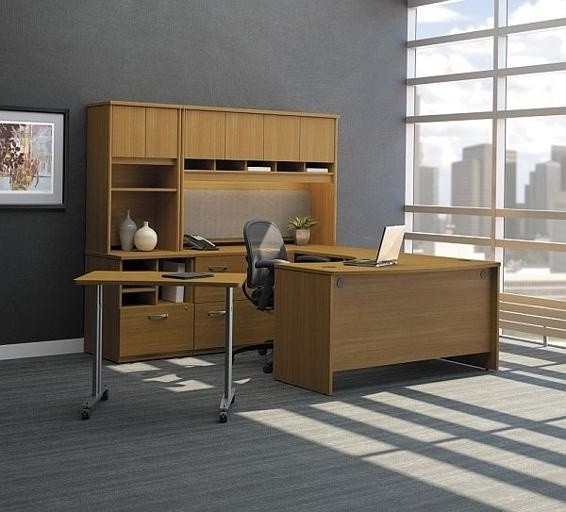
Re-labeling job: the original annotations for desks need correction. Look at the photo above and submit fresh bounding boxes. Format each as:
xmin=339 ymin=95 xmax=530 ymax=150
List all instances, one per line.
xmin=84 ymin=241 xmax=501 ymax=397
xmin=73 ymin=269 xmax=248 ymax=423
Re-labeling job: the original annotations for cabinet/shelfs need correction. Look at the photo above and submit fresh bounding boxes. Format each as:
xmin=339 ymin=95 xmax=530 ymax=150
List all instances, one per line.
xmin=83 ymin=102 xmax=339 ymax=192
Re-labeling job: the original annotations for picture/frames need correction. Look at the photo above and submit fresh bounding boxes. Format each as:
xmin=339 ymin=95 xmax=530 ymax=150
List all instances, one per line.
xmin=0 ymin=104 xmax=71 ymax=213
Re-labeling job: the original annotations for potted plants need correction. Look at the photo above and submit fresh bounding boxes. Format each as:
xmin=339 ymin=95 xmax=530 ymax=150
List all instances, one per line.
xmin=286 ymin=214 xmax=318 ymax=245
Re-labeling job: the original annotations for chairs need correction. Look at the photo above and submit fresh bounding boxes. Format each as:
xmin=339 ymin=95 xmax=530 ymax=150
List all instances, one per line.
xmin=233 ymin=220 xmax=333 ymax=373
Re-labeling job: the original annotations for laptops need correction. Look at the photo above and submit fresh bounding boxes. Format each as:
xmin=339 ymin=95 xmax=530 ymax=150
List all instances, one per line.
xmin=343 ymin=225 xmax=407 ymax=268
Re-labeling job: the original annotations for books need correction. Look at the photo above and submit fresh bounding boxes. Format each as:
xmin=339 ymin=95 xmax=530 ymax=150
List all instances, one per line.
xmin=160 ymin=260 xmax=215 ymax=304
xmin=306 ymin=168 xmax=328 ymax=174
xmin=248 ymin=167 xmax=271 ymax=172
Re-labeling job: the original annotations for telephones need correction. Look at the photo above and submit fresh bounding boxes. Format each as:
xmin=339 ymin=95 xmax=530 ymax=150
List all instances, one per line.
xmin=184 ymin=234 xmax=217 ymax=250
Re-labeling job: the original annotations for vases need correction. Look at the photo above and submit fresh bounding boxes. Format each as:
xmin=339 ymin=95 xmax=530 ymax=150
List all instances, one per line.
xmin=118 ymin=208 xmax=158 ymax=251
